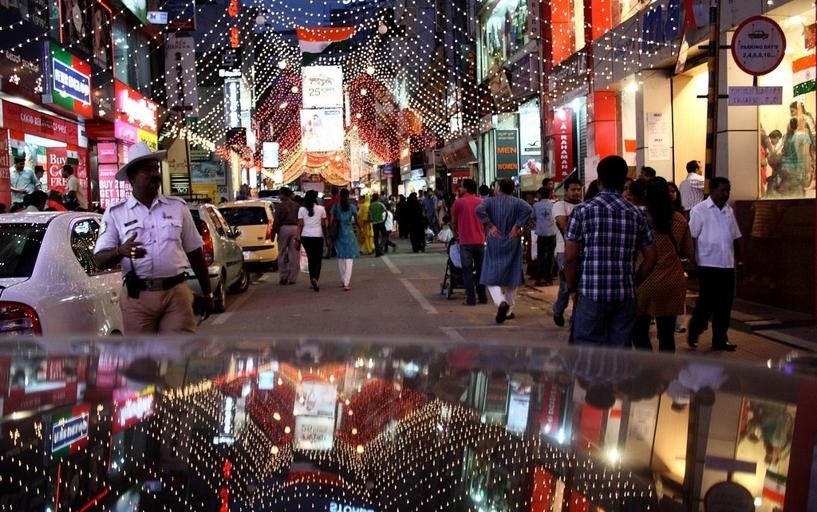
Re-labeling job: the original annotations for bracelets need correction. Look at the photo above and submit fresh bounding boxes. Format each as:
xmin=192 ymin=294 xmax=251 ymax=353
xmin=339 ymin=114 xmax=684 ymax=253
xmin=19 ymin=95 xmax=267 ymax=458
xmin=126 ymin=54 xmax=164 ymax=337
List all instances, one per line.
xmin=116 ymin=245 xmax=123 ymax=259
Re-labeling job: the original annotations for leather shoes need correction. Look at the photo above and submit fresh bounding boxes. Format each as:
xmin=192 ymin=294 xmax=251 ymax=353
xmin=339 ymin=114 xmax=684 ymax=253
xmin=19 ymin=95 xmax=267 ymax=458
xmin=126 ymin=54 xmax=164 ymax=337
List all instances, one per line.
xmin=496 ymin=300 xmax=509 ymax=324
xmin=552 ymin=302 xmax=565 ymax=327
xmin=278 ymin=279 xmax=287 ymax=285
xmin=287 ymin=281 xmax=295 ymax=285
xmin=673 ymin=325 xmax=687 ymax=333
xmin=687 ymin=334 xmax=699 ymax=348
xmin=711 ymin=341 xmax=737 ymax=350
xmin=506 ymin=312 xmax=515 ymax=319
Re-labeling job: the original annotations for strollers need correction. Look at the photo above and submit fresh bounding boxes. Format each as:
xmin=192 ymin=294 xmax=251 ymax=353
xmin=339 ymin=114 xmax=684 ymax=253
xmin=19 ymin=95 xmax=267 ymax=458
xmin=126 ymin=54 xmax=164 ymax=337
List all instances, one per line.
xmin=440 ymin=236 xmax=477 ymax=299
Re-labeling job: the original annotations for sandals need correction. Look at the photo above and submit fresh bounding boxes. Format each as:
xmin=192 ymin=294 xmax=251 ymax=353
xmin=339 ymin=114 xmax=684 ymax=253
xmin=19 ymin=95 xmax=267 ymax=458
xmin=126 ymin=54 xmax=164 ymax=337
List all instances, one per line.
xmin=392 ymin=241 xmax=397 ymax=252
xmin=462 ymin=299 xmax=476 ymax=305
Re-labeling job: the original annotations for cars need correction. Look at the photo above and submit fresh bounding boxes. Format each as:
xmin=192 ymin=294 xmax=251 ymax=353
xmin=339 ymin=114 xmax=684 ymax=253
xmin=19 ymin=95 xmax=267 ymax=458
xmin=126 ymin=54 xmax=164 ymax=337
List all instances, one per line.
xmin=183 ymin=204 xmax=248 ymax=313
xmin=0 ymin=210 xmax=122 ymax=337
xmin=217 ymin=200 xmax=278 ymax=271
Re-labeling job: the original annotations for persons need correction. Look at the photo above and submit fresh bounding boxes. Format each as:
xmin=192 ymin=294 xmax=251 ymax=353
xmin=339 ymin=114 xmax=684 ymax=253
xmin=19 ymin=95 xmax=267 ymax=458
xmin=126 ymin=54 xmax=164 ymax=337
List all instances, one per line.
xmin=623 ymin=177 xmax=636 ymax=206
xmin=790 ymin=102 xmax=817 ymax=178
xmin=328 ymin=187 xmax=361 ymax=291
xmin=759 ymin=122 xmax=771 ymax=199
xmin=390 ymin=196 xmax=397 ymax=232
xmin=9 ymin=156 xmax=42 ymax=203
xmin=450 ymin=179 xmax=488 ymax=306
xmin=9 ymin=201 xmax=23 ymax=213
xmin=294 ymin=195 xmax=303 ymax=269
xmin=382 ymin=203 xmax=397 ymax=251
xmin=422 ymin=187 xmax=440 ymax=235
xmin=476 ymin=177 xmax=532 ymax=324
xmin=20 ymin=190 xmax=48 ymax=211
xmin=629 ymin=177 xmax=654 ymax=226
xmin=686 ymin=178 xmax=751 ymax=350
xmin=34 ymin=165 xmax=45 ymax=180
xmin=552 ymin=178 xmax=584 ymax=328
xmin=236 ymin=190 xmax=248 ymax=201
xmin=368 ymin=193 xmax=388 ymax=257
xmin=438 ymin=191 xmax=449 ymax=229
xmin=44 ymin=190 xmax=62 ymax=212
xmin=678 ymin=160 xmax=705 ymax=279
xmin=631 ymin=176 xmax=693 ymax=353
xmin=315 ymin=191 xmax=322 ymax=205
xmin=584 ymin=180 xmax=599 ymax=201
xmin=218 ymin=196 xmax=226 ymax=204
xmin=533 ymin=177 xmax=558 ymax=203
xmin=394 ymin=195 xmax=409 ymax=239
xmin=6 ymin=339 xmax=729 ymax=414
xmin=765 ymin=129 xmax=782 ymax=198
xmin=62 ymin=165 xmax=88 ymax=211
xmin=479 ymin=185 xmax=490 ymax=200
xmin=92 ymin=141 xmax=214 ymax=337
xmin=324 ymin=187 xmax=341 ymax=259
xmin=406 ymin=193 xmax=426 ymax=253
xmin=270 ymin=187 xmax=300 ymax=285
xmin=669 ymin=182 xmax=688 ymax=220
xmin=417 ymin=190 xmax=425 ymax=204
xmin=379 ymin=191 xmax=388 ymax=205
xmin=297 ymin=189 xmax=328 ymax=292
xmin=564 ymin=154 xmax=656 ymax=349
xmin=530 ymin=187 xmax=558 ymax=285
xmin=356 ymin=194 xmax=375 ymax=255
xmin=780 ymin=118 xmax=810 ymax=198
xmin=639 ymin=167 xmax=657 ymax=179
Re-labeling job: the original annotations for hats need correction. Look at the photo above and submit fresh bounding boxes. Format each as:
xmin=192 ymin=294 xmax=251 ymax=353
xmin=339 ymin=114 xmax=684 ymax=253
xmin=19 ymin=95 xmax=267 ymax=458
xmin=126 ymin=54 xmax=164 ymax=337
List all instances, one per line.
xmin=34 ymin=166 xmax=45 ymax=174
xmin=115 ymin=140 xmax=167 ymax=181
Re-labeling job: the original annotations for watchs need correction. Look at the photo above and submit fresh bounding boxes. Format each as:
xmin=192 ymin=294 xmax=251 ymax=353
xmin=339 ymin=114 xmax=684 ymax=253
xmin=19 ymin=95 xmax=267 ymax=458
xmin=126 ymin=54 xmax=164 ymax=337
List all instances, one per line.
xmin=567 ymin=286 xmax=576 ymax=293
xmin=204 ymin=293 xmax=213 ymax=299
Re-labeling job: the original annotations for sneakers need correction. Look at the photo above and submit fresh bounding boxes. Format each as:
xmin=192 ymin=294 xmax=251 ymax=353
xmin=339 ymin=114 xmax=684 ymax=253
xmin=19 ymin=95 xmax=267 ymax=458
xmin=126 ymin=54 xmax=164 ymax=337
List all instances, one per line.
xmin=311 ymin=279 xmax=320 ymax=292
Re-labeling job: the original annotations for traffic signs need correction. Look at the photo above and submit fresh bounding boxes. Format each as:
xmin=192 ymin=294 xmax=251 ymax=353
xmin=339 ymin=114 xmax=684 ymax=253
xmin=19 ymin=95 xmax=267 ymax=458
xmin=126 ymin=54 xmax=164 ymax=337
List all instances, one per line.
xmin=732 ymin=15 xmax=786 ymax=75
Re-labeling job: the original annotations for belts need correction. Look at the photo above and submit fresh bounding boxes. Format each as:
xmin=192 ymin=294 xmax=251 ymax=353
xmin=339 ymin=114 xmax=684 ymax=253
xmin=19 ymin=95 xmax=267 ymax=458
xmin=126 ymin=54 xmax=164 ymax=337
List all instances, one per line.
xmin=123 ymin=271 xmax=189 ymax=291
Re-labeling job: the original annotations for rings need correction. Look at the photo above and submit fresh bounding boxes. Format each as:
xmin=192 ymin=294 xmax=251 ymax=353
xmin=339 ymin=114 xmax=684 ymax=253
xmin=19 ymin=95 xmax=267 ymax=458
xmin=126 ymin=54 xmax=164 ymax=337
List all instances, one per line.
xmin=131 ymin=246 xmax=137 ymax=251
xmin=130 ymin=252 xmax=135 ymax=255
xmin=132 ymin=256 xmax=135 ymax=258
